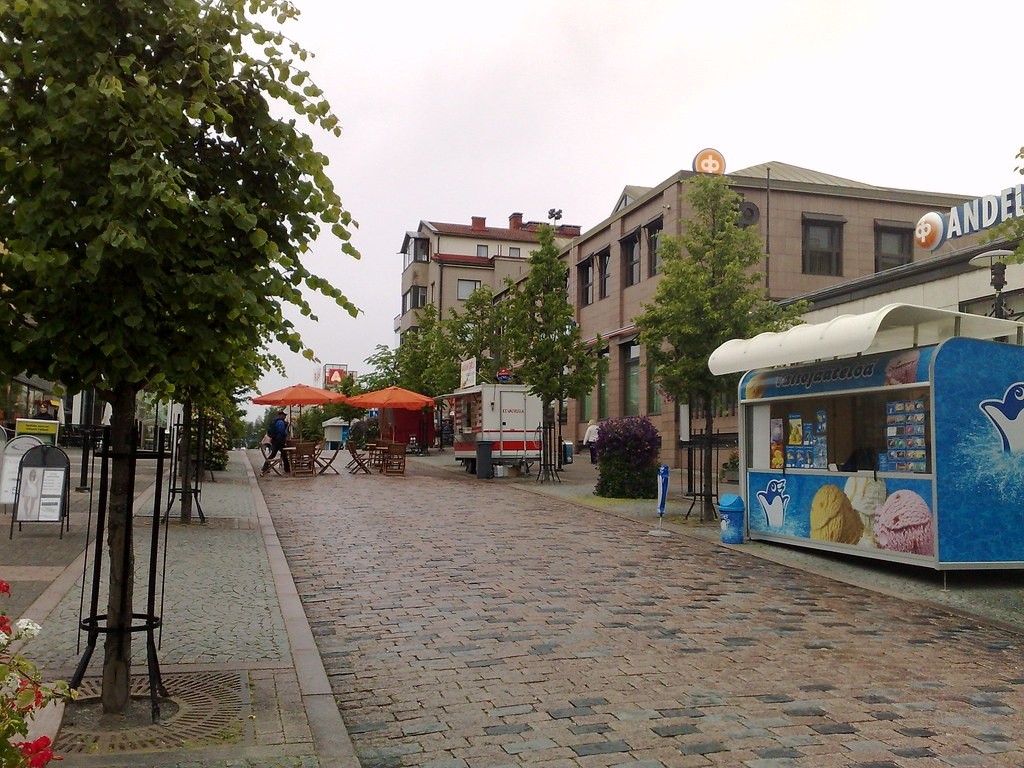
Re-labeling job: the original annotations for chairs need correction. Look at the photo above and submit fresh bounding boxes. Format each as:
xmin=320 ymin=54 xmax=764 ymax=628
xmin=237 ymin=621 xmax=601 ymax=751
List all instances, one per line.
xmin=258 ymin=441 xmax=283 ymax=477
xmin=318 ymin=442 xmax=344 ymax=475
xmin=314 ymin=438 xmax=327 ymax=467
xmin=292 ymin=443 xmax=316 ymax=478
xmin=346 ymin=439 xmax=407 ymax=476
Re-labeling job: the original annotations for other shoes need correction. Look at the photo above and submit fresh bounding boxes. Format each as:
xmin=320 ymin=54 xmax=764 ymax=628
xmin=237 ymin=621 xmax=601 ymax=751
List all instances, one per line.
xmin=262 ymin=469 xmax=270 ymax=473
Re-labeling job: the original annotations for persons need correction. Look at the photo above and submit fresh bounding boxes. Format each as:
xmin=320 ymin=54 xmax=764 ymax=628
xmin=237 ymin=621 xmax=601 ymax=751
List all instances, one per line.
xmin=35 ymin=403 xmax=53 ymax=419
xmin=259 ymin=412 xmax=289 ymax=476
xmin=434 ymin=423 xmax=440 ymax=442
xmin=583 ymin=420 xmax=600 ymax=463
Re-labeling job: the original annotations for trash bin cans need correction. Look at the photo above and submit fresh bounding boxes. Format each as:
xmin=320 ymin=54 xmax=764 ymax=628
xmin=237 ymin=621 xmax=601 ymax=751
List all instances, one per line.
xmin=563 ymin=440 xmax=573 ymax=464
xmin=475 ymin=441 xmax=494 ymax=479
xmin=718 ymin=494 xmax=745 ymax=544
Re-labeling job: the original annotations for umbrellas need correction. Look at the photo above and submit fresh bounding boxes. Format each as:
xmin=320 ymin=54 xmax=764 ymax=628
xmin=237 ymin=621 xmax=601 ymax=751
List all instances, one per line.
xmin=253 ymin=384 xmax=346 ymax=442
xmin=344 ymin=386 xmax=435 ymax=441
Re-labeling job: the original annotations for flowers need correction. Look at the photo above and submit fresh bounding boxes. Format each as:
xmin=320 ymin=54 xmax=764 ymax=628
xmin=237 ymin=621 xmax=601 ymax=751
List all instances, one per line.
xmin=0 ymin=580 xmax=79 ymax=768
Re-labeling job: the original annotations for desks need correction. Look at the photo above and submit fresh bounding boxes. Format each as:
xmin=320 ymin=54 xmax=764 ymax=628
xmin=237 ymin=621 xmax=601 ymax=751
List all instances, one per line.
xmin=284 ymin=445 xmax=319 ymax=472
xmin=362 ymin=447 xmax=388 ymax=470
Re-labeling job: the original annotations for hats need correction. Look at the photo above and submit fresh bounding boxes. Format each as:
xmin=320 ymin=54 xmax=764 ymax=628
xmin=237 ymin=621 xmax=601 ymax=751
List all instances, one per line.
xmin=588 ymin=419 xmax=597 ymax=425
xmin=278 ymin=412 xmax=287 ymax=415
